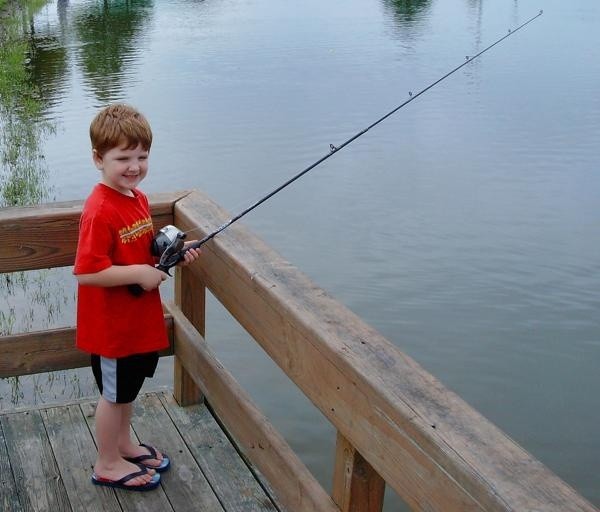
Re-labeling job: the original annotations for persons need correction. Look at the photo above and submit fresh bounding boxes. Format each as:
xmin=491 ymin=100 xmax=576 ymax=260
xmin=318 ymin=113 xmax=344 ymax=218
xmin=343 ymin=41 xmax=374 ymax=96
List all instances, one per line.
xmin=73 ymin=103 xmax=202 ymax=491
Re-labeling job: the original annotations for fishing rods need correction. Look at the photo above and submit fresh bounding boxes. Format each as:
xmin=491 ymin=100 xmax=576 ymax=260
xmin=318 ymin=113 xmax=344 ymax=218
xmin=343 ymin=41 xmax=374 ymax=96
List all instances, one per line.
xmin=130 ymin=12 xmax=544 ymax=294
